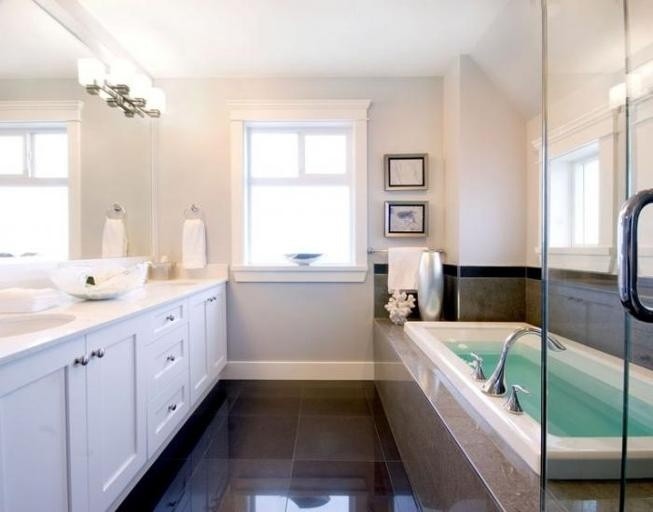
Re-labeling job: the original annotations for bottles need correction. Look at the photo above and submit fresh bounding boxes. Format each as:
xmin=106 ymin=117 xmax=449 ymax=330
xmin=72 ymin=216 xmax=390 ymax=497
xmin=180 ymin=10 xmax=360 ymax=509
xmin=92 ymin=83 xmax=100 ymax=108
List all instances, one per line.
xmin=157 ymin=256 xmax=171 ymax=279
xmin=418 ymin=249 xmax=446 ymax=321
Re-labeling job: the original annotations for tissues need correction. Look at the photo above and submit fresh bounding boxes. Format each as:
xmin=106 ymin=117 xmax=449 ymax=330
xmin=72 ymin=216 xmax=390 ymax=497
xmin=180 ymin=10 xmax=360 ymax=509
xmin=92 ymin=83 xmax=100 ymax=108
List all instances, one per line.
xmin=150 ymin=256 xmax=177 ymax=279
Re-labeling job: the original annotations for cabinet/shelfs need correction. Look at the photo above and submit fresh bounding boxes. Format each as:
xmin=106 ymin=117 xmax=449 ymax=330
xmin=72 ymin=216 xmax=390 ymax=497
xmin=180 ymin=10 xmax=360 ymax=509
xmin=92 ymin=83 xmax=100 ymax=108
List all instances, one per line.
xmin=189 ymin=284 xmax=226 ymax=407
xmin=0 ymin=319 xmax=145 ymax=512
xmin=146 ymin=299 xmax=187 ymax=461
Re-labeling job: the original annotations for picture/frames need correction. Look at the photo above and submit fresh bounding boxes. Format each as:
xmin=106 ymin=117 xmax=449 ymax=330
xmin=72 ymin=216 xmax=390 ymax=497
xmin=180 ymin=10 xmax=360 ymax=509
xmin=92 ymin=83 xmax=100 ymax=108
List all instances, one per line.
xmin=382 ymin=152 xmax=428 ymax=191
xmin=383 ymin=200 xmax=429 ymax=239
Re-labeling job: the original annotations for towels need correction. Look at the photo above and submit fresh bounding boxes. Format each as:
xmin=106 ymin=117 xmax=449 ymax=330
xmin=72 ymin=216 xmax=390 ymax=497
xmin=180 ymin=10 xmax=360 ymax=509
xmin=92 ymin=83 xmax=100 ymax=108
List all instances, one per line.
xmin=100 ymin=216 xmax=128 ymax=259
xmin=180 ymin=220 xmax=207 ymax=268
xmin=387 ymin=247 xmax=429 ymax=294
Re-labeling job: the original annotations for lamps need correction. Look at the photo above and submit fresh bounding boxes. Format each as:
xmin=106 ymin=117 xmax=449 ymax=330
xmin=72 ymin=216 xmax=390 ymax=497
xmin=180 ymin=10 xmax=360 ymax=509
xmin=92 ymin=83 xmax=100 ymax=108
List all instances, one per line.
xmin=104 ymin=61 xmax=167 ymax=119
xmin=79 ymin=57 xmax=144 ymax=120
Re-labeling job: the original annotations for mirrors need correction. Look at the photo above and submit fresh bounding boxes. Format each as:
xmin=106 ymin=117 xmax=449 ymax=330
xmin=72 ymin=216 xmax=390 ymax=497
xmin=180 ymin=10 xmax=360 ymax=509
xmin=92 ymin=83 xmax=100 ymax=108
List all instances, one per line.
xmin=0 ymin=0 xmax=155 ymax=261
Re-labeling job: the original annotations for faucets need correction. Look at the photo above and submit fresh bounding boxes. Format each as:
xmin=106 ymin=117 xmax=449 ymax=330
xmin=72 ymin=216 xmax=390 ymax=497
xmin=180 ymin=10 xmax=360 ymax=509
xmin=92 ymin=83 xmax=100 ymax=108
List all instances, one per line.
xmin=481 ymin=326 xmax=566 ymax=395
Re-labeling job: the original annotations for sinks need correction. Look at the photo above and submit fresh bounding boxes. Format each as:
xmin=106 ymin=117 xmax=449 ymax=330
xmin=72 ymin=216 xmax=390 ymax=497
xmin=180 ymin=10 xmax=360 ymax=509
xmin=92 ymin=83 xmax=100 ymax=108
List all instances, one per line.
xmin=43 ymin=260 xmax=148 ymax=300
xmin=0 ymin=314 xmax=82 ymax=336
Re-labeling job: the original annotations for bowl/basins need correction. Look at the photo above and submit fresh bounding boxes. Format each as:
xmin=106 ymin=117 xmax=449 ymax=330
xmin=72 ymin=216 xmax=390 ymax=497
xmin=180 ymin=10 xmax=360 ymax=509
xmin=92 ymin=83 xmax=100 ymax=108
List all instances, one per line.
xmin=286 ymin=253 xmax=322 ymax=266
xmin=42 ymin=255 xmax=153 ymax=298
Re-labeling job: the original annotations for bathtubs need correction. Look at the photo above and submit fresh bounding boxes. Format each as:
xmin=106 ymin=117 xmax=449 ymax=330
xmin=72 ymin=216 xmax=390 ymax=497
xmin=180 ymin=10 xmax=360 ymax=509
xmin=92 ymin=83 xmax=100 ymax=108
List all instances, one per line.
xmin=404 ymin=320 xmax=652 ymax=481
